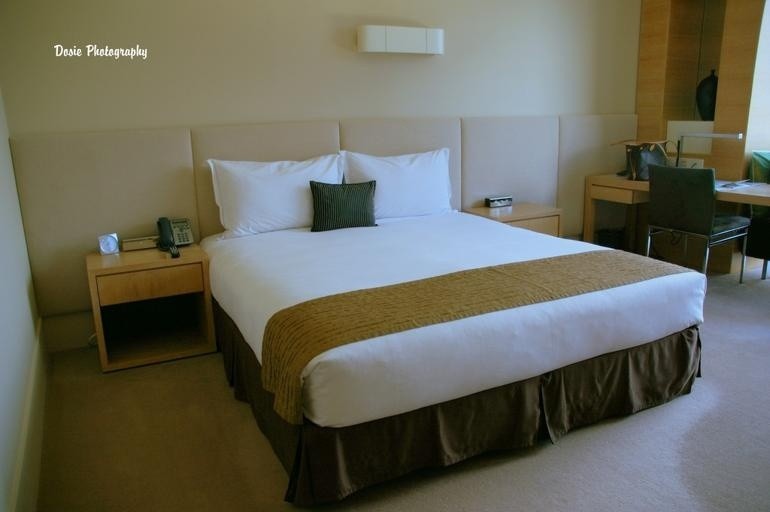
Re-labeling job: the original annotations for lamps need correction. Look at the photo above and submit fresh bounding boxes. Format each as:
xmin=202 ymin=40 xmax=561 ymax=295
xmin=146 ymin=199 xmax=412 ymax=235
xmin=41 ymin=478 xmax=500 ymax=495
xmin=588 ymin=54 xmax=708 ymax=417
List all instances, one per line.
xmin=360 ymin=25 xmax=444 ymax=55
xmin=679 ymin=133 xmax=742 ymax=162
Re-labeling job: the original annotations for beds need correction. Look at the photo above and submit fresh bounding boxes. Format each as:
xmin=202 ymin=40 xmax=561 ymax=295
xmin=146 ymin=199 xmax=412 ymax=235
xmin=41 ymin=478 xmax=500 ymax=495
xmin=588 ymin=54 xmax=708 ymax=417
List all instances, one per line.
xmin=200 ymin=212 xmax=709 ymax=512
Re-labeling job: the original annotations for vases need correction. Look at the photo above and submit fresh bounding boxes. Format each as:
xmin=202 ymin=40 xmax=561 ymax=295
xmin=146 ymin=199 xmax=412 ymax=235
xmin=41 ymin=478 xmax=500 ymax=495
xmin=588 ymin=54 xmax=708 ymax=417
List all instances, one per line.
xmin=695 ymin=69 xmax=718 ymax=121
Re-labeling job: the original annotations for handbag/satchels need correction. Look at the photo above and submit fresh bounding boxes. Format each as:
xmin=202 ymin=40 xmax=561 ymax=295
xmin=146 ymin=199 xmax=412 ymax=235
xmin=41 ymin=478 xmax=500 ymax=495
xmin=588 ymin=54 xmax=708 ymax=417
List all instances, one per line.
xmin=625 ymin=140 xmax=667 ymax=181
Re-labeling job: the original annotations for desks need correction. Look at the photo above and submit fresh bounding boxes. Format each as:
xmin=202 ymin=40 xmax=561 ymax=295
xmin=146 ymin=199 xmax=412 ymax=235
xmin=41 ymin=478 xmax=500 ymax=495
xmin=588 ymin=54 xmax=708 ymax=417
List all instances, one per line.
xmin=583 ymin=171 xmax=770 ymax=242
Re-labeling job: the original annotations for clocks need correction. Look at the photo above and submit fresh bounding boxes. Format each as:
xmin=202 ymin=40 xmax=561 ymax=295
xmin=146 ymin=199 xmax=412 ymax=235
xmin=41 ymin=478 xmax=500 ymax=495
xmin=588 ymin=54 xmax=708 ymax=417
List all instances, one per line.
xmin=97 ymin=233 xmax=120 ymax=255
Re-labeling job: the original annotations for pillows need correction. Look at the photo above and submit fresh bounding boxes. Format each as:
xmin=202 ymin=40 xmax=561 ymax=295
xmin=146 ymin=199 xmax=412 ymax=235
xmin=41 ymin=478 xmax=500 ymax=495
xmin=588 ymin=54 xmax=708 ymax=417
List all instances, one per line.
xmin=340 ymin=147 xmax=455 ymax=217
xmin=209 ymin=154 xmax=346 ymax=238
xmin=309 ymin=180 xmax=378 ymax=231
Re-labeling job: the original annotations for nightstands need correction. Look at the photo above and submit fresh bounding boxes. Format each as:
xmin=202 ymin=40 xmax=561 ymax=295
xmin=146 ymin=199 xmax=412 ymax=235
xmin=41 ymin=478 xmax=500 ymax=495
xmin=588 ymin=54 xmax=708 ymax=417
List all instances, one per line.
xmin=83 ymin=244 xmax=217 ymax=373
xmin=464 ymin=204 xmax=562 ymax=238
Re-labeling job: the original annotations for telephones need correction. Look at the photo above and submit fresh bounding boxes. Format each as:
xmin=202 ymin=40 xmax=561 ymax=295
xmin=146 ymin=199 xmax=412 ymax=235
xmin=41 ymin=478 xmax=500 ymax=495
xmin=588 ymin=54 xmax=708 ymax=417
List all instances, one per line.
xmin=157 ymin=217 xmax=194 ymax=248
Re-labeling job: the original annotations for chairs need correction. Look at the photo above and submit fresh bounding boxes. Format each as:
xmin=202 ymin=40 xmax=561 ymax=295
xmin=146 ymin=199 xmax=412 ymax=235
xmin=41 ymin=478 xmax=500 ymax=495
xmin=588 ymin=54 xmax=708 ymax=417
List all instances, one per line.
xmin=645 ymin=164 xmax=750 ymax=283
